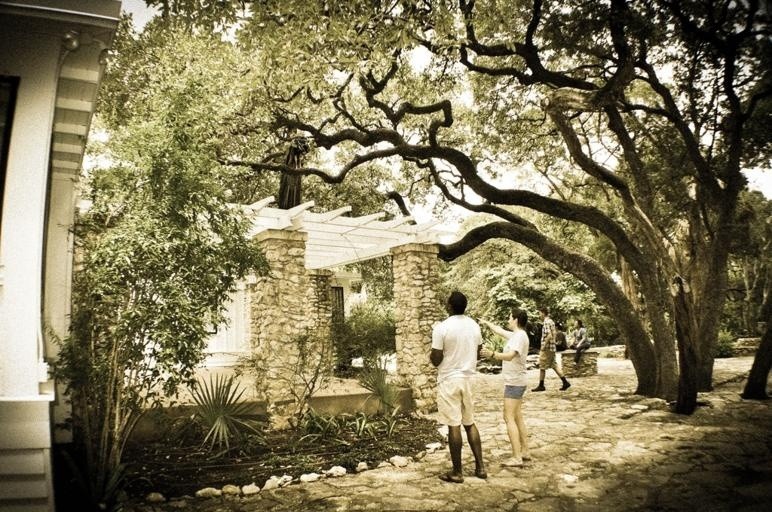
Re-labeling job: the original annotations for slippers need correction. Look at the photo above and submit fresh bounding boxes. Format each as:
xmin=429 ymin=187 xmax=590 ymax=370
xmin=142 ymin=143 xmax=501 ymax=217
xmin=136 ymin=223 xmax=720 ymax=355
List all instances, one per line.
xmin=475 ymin=469 xmax=487 ymax=479
xmin=438 ymin=473 xmax=464 ymax=483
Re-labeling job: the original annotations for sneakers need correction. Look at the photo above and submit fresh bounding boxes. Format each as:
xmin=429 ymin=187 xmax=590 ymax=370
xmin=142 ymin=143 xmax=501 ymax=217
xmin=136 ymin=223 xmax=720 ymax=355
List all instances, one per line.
xmin=531 ymin=386 xmax=545 ymax=391
xmin=500 ymin=453 xmax=532 ymax=467
xmin=559 ymin=382 xmax=571 ymax=390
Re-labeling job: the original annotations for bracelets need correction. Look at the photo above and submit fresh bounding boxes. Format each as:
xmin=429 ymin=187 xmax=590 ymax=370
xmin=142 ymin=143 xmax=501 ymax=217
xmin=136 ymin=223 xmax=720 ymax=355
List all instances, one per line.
xmin=491 ymin=352 xmax=495 ymax=358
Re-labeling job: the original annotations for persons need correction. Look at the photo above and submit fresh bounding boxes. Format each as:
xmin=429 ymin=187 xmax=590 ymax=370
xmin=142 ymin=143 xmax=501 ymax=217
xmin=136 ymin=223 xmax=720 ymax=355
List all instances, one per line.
xmin=532 ymin=307 xmax=571 ymax=391
xmin=476 ymin=309 xmax=531 ymax=467
xmin=429 ymin=291 xmax=487 ymax=483
xmin=570 ymin=320 xmax=591 ymax=365
xmin=555 ymin=323 xmax=569 ymax=351
